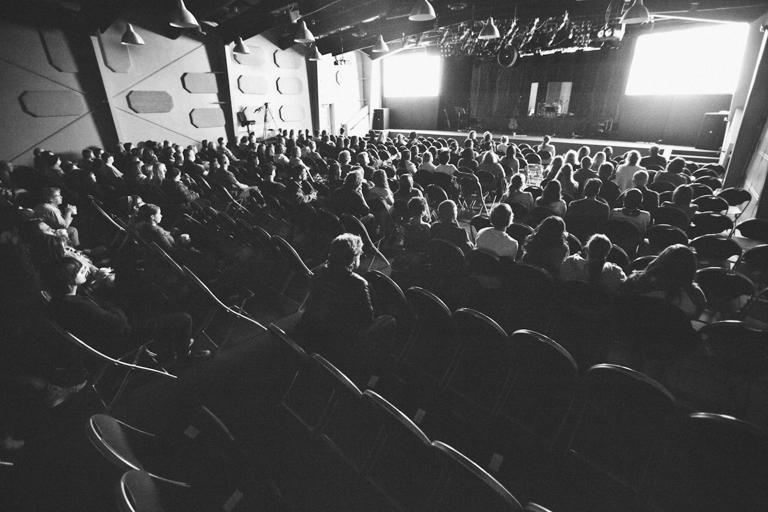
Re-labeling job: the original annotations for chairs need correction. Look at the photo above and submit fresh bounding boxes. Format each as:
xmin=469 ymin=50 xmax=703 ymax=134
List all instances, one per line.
xmin=1 ymin=143 xmax=768 ymax=512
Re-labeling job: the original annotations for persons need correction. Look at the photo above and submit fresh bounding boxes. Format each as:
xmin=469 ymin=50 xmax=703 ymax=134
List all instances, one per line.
xmin=0 ymin=127 xmax=704 ymax=376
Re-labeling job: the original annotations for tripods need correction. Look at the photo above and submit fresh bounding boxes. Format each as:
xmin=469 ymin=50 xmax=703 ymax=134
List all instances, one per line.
xmin=263 ymin=107 xmax=279 ymax=139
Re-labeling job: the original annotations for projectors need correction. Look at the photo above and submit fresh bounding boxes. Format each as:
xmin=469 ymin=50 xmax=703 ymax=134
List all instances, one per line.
xmin=334 ymin=60 xmax=351 ymax=65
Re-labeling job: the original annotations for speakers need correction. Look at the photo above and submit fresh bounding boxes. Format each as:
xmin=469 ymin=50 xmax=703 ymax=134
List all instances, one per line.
xmin=694 ymin=112 xmax=728 ymax=149
xmin=373 ymin=108 xmax=390 ymax=130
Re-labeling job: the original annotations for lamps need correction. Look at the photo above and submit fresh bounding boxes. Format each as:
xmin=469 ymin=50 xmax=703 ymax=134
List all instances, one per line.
xmin=310 ymin=47 xmax=322 ymax=61
xmin=293 ymin=21 xmax=315 ymax=44
xmin=279 ymin=6 xmax=300 ymax=25
xmin=408 ymin=2 xmax=436 ymax=22
xmin=478 ymin=16 xmax=499 ymax=39
xmin=599 ymin=30 xmax=625 ymax=42
xmin=351 ymin=25 xmax=367 ymax=38
xmin=372 ymin=35 xmax=390 ymax=53
xmin=233 ymin=36 xmax=249 ymax=56
xmin=168 ymin=2 xmax=201 ymax=29
xmin=120 ymin=24 xmax=143 ymax=46
